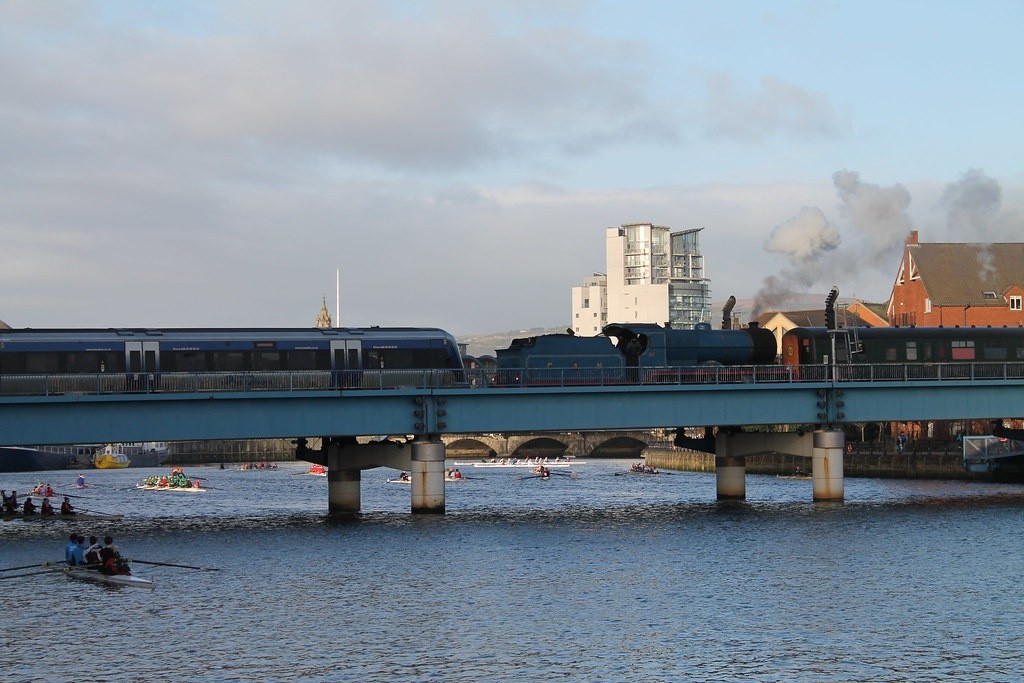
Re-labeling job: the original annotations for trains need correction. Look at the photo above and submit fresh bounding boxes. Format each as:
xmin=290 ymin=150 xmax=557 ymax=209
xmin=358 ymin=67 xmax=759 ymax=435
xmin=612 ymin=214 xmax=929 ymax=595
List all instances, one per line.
xmin=0 ymin=316 xmax=1024 ymax=393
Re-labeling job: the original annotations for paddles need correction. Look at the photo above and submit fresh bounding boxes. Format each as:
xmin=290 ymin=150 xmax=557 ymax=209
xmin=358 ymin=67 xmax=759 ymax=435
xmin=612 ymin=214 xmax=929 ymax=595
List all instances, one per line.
xmin=0 ymin=560 xmax=67 ymax=572
xmin=40 ymin=491 xmax=85 ymax=499
xmin=550 ymin=469 xmax=586 ymax=480
xmin=0 ymin=562 xmax=104 ymax=579
xmin=123 ymin=559 xmax=221 ymax=571
xmin=84 ymin=482 xmax=113 ymax=488
xmin=188 ymin=476 xmax=207 ymax=480
xmin=19 ymin=503 xmax=112 ymax=516
xmin=55 ymin=482 xmax=75 ymax=488
xmin=199 ymin=486 xmax=223 ymax=490
xmin=114 ymin=485 xmax=142 ymax=492
xmin=515 ymin=475 xmax=543 ymax=480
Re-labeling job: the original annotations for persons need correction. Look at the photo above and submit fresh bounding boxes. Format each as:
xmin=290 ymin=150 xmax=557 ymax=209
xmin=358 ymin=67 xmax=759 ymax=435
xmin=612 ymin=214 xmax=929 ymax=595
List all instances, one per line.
xmin=630 ymin=463 xmax=656 ymax=473
xmin=143 ymin=468 xmax=201 ymax=489
xmin=958 ymin=430 xmax=966 ymax=448
xmin=219 ymin=462 xmax=279 ymax=468
xmin=896 ymin=432 xmax=906 ymax=453
xmin=482 ymin=456 xmax=570 ymax=476
xmin=399 ymin=466 xmax=461 ymax=480
xmin=790 ymin=467 xmax=801 ymax=476
xmin=0 ymin=474 xmax=132 ymax=576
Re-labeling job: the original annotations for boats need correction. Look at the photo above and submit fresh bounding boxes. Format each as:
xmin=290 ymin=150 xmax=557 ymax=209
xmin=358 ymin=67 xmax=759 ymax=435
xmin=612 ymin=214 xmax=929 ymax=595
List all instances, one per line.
xmin=445 ymin=478 xmax=463 ymax=481
xmin=388 ymin=476 xmax=412 ymax=483
xmin=775 ymin=474 xmax=813 ymax=480
xmin=300 ymin=473 xmax=328 ymax=477
xmin=2 ymin=513 xmax=123 ymax=522
xmin=135 ymin=483 xmax=206 ymax=493
xmin=43 ymin=560 xmax=156 ymax=589
xmin=454 ymin=459 xmax=587 ymax=467
xmin=92 ymin=443 xmax=132 ymax=470
